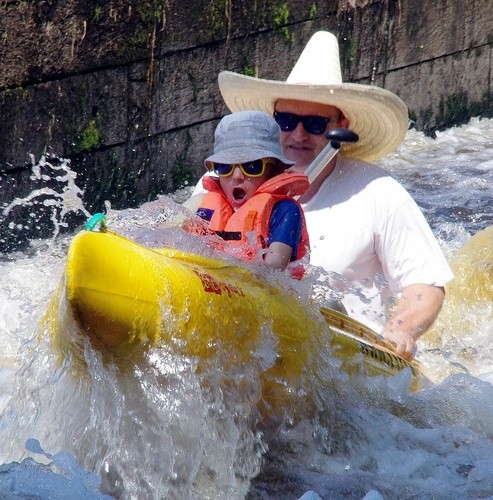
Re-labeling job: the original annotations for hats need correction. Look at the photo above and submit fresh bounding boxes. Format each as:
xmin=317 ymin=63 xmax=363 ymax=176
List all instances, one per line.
xmin=216 ymin=30 xmax=409 ymax=162
xmin=204 ymin=110 xmax=296 ymax=173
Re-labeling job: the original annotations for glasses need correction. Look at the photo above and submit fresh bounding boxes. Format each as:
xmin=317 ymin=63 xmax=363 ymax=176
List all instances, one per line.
xmin=273 ymin=109 xmax=341 ymax=136
xmin=211 ymin=159 xmax=278 ymax=177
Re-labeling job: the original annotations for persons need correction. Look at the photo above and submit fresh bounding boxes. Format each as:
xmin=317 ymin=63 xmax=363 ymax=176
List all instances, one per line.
xmin=183 ymin=109 xmax=310 ymax=282
xmin=157 ymin=30 xmax=457 ymax=369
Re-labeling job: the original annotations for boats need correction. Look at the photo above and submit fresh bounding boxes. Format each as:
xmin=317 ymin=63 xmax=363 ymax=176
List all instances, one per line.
xmin=38 ymin=213 xmax=493 ymax=451
